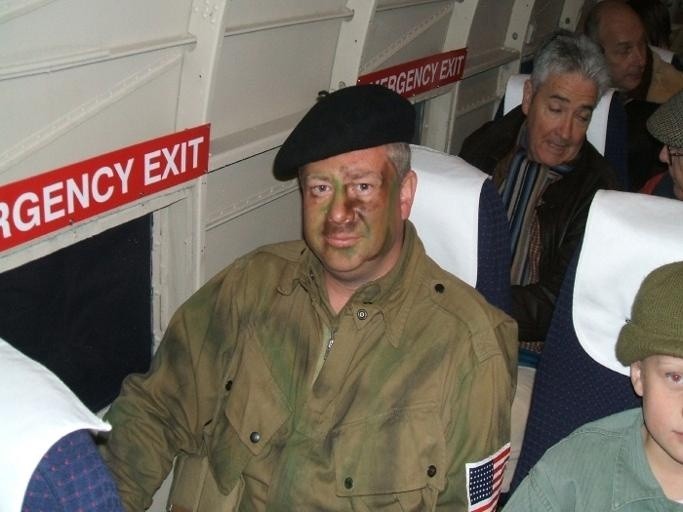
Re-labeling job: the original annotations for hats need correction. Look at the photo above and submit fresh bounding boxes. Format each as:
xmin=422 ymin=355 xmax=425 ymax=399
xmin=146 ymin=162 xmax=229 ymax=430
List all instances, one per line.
xmin=644 ymin=89 xmax=682 ymax=150
xmin=613 ymin=259 xmax=683 ymax=368
xmin=269 ymin=83 xmax=417 ymax=183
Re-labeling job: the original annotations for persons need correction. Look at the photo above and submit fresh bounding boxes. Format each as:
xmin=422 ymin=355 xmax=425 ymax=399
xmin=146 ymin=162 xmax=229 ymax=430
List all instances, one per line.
xmin=94 ymin=83 xmax=519 ymax=511
xmin=584 ymin=0 xmax=681 ymax=105
xmin=502 ymin=260 xmax=682 ymax=511
xmin=458 ymin=33 xmax=620 ymax=506
xmin=637 ymin=88 xmax=683 ymax=202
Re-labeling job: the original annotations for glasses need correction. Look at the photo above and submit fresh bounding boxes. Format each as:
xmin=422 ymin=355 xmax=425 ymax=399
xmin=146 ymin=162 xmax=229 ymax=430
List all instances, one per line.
xmin=665 ymin=144 xmax=682 ymax=166
